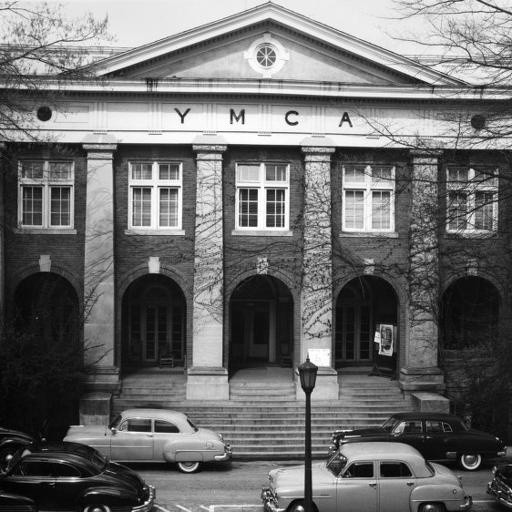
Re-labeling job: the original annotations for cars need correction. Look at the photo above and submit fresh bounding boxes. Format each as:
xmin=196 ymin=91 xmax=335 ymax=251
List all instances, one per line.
xmin=486 ymin=463 xmax=512 ymax=510
xmin=0 ymin=425 xmax=33 ymax=469
xmin=328 ymin=412 xmax=508 ymax=472
xmin=0 ymin=440 xmax=156 ymax=512
xmin=61 ymin=408 xmax=232 ymax=473
xmin=261 ymin=442 xmax=473 ymax=512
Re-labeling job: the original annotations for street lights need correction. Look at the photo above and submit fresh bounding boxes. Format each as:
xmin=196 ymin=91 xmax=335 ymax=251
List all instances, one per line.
xmin=298 ymin=353 xmax=318 ymax=512
